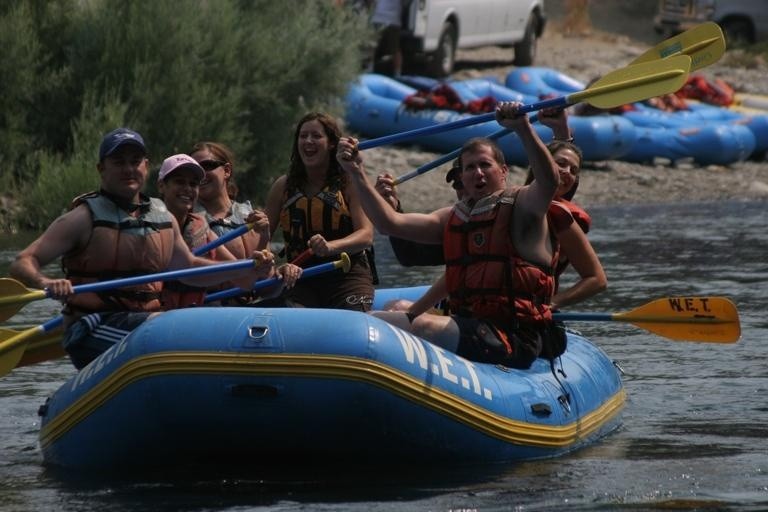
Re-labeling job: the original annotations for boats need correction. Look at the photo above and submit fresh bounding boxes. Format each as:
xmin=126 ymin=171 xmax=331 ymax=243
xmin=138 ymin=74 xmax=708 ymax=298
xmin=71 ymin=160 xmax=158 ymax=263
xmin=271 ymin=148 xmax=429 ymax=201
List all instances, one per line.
xmin=37 ymin=284 xmax=625 ymax=484
xmin=503 ymin=66 xmax=768 ymax=164
xmin=442 ymin=77 xmax=757 ymax=168
xmin=339 ymin=71 xmax=638 ymax=165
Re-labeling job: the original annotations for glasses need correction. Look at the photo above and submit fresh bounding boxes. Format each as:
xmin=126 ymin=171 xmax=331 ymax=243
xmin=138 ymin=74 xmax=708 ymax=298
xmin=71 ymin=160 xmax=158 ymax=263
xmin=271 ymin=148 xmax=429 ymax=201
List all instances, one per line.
xmin=453 ymin=181 xmax=463 ymax=189
xmin=199 ymin=160 xmax=226 ymax=170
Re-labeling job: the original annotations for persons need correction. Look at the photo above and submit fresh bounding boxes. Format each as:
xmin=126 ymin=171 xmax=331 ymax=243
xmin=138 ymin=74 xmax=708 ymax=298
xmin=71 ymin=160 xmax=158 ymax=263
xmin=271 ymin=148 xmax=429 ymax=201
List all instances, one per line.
xmin=190 ymin=140 xmax=303 ymax=306
xmin=264 ymin=111 xmax=374 ymax=313
xmin=156 ymin=154 xmax=271 ymax=311
xmin=9 ymin=128 xmax=275 ymax=371
xmin=336 ymin=96 xmax=608 ymax=370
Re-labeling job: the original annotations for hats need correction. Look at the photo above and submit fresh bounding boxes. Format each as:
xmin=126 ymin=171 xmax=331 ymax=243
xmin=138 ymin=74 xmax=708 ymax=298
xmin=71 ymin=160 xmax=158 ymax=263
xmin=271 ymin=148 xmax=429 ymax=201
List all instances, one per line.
xmin=446 ymin=159 xmax=463 ymax=182
xmin=99 ymin=129 xmax=146 ymax=156
xmin=159 ymin=153 xmax=206 ymax=183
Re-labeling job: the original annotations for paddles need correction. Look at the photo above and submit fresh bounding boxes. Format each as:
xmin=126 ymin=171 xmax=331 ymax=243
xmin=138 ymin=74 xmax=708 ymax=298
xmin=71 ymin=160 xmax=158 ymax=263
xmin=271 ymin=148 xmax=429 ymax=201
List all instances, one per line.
xmin=392 ymin=21 xmax=727 ymax=186
xmin=0 ymin=251 xmax=351 ymax=367
xmin=354 ymin=55 xmax=693 ymax=153
xmin=0 ymin=258 xmax=256 ymax=325
xmin=0 ymin=221 xmax=255 ymax=376
xmin=550 ymin=298 xmax=740 ymax=343
xmin=288 ymin=247 xmax=314 ymax=266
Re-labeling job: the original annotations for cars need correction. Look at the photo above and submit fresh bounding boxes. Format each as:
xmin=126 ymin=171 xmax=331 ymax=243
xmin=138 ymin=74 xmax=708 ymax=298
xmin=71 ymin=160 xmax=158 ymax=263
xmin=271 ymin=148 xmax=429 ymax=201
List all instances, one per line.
xmin=652 ymin=2 xmax=768 ymax=50
xmin=342 ymin=1 xmax=547 ymax=78
